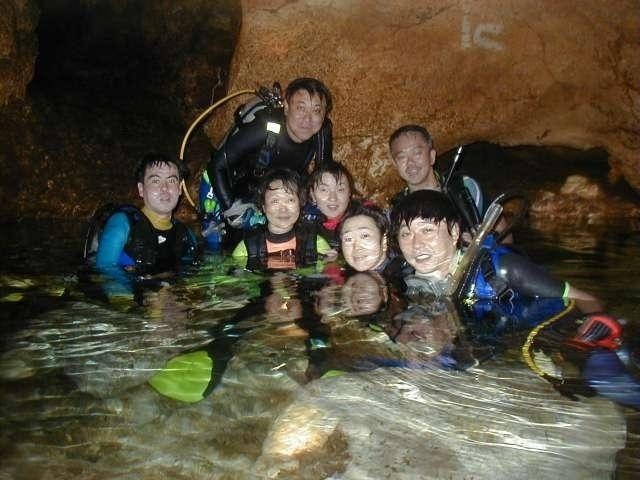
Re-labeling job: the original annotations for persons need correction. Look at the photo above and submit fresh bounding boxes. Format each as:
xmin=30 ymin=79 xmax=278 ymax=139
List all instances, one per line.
xmin=351 ymin=189 xmax=640 ymax=410
xmin=149 ymin=167 xmax=349 ymax=402
xmin=261 ymin=288 xmax=340 ymax=338
xmin=81 ymin=148 xmax=199 ymax=318
xmin=335 ymin=271 xmax=391 ymax=322
xmin=389 ymin=124 xmax=483 ymax=218
xmin=395 ymin=299 xmax=479 ymax=363
xmin=201 ymin=78 xmax=333 ymax=250
xmin=334 ymin=205 xmax=393 ymax=277
